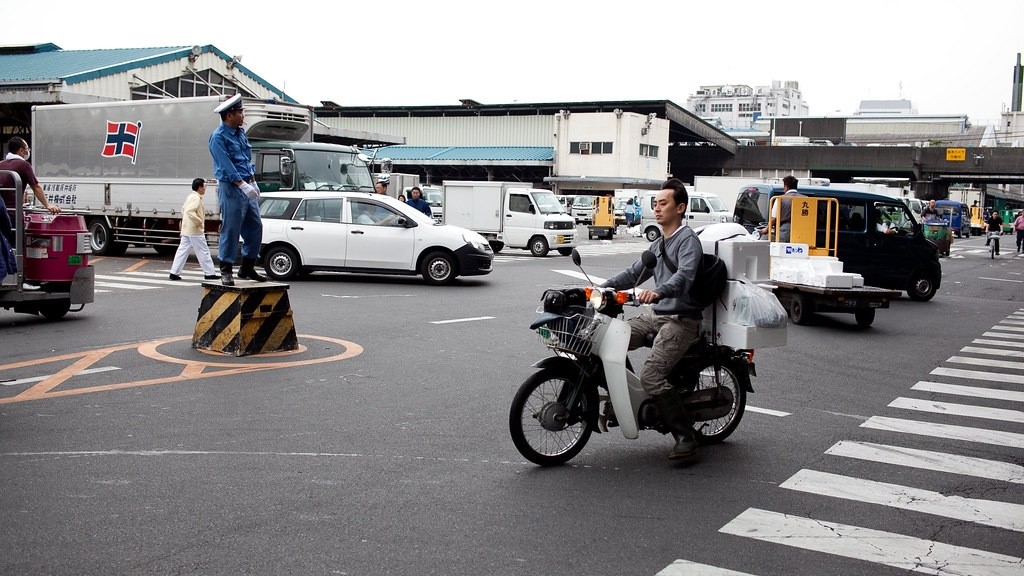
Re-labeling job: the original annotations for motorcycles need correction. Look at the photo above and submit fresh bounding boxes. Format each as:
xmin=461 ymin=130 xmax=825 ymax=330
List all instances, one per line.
xmin=508 ymin=247 xmax=758 ymax=467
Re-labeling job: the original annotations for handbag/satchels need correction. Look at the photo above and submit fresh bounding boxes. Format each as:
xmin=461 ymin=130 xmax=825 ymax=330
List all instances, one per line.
xmin=541 ymin=288 xmax=587 ymax=345
xmin=689 ymin=252 xmax=727 ymax=307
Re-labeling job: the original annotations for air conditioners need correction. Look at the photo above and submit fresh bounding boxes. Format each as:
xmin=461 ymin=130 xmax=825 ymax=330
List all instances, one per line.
xmin=579 ymin=143 xmax=590 ymax=151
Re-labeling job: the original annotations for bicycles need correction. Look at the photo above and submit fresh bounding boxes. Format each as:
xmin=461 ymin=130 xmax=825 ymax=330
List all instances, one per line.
xmin=982 ymin=231 xmax=1003 ymax=259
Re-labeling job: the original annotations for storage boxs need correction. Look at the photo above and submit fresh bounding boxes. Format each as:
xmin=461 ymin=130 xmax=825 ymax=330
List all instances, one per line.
xmin=699 ymin=237 xmax=865 ymax=349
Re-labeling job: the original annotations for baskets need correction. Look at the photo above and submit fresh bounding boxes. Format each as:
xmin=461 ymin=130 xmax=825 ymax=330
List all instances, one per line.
xmin=987 ymin=232 xmax=1000 ymax=239
xmin=535 ymin=305 xmax=607 ymax=357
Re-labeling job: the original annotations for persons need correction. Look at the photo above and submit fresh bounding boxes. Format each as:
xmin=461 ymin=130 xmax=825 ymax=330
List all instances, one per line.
xmin=633 ymin=196 xmax=640 ymax=207
xmin=398 ymin=195 xmax=406 ymax=203
xmin=593 ymin=178 xmax=705 ymax=461
xmin=375 ymin=181 xmax=388 ymax=195
xmin=0 ymin=137 xmax=62 ymax=282
xmin=985 ymin=210 xmax=1004 ymax=255
xmin=606 ymin=194 xmax=614 ymax=213
xmin=921 ymin=199 xmax=939 ymax=222
xmin=970 ymin=201 xmax=979 ymax=218
xmin=759 ymin=173 xmax=809 ymax=243
xmin=168 ymin=178 xmax=223 ymax=281
xmin=406 ymin=187 xmax=431 ymax=217
xmin=1013 ymin=209 xmax=1024 ymax=254
xmin=208 ymin=92 xmax=269 ymax=285
xmin=873 ymin=206 xmax=897 ymax=235
xmin=356 ymin=203 xmax=398 ymax=225
xmin=624 ymin=198 xmax=636 ymax=229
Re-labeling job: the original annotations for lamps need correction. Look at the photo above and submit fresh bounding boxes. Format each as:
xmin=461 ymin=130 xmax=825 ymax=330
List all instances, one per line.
xmin=614 ymin=108 xmax=623 ymax=119
xmin=226 ymin=54 xmax=243 ymax=70
xmin=641 ymin=122 xmax=652 ymax=135
xmin=189 ymin=45 xmax=201 ymax=63
xmin=560 ymin=110 xmax=570 ymax=120
xmin=645 ymin=112 xmax=657 ymax=123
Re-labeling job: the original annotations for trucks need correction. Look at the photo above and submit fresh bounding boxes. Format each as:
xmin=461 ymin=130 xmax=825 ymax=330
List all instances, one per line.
xmin=25 ymin=94 xmax=394 ymax=257
xmin=439 ymin=180 xmax=579 ymax=259
xmin=367 ymin=171 xmax=1019 ymax=328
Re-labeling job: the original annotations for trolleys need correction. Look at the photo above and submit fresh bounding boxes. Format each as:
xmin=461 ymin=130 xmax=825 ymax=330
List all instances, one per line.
xmin=921 ymin=218 xmax=956 ymax=257
xmin=1 ymin=168 xmax=96 ymax=322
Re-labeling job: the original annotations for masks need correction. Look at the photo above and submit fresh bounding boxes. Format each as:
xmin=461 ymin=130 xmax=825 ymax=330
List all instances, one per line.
xmin=22 ymin=149 xmax=31 ymax=161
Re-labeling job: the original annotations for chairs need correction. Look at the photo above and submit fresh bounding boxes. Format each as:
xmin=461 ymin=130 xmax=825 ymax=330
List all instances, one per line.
xmin=848 ymin=213 xmax=864 ymax=231
xmin=943 ymin=210 xmax=948 ymax=215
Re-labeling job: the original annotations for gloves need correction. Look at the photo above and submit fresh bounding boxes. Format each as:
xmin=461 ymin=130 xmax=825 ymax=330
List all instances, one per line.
xmin=238 ymin=182 xmax=258 ymax=200
xmin=250 ymin=181 xmax=260 ymax=196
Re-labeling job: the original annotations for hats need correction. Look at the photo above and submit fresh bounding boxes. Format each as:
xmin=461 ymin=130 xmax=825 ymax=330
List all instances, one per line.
xmin=214 ymin=93 xmax=247 ymax=115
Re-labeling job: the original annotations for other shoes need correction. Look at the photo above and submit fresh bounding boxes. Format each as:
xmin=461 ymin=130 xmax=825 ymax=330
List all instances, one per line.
xmin=23 ymin=281 xmax=41 ymax=290
xmin=985 ymin=243 xmax=989 ymax=246
xmin=170 ymin=273 xmax=181 ymax=280
xmin=205 ymin=274 xmax=221 ymax=280
xmin=996 ymin=252 xmax=1000 ymax=255
xmin=1017 ymin=246 xmax=1020 ymax=252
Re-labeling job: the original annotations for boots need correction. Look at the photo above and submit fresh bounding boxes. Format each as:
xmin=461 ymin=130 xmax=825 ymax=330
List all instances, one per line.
xmin=220 ymin=261 xmax=235 ymax=285
xmin=652 ymin=389 xmax=700 ymax=459
xmin=238 ymin=255 xmax=268 ymax=282
xmin=608 ymin=356 xmax=634 ymax=427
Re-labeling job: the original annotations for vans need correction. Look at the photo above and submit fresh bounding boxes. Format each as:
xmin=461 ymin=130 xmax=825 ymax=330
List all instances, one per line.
xmin=731 ymin=184 xmax=943 ymax=303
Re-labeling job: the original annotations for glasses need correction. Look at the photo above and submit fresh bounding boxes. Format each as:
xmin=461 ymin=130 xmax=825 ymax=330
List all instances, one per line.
xmin=21 ymin=147 xmax=31 ymax=152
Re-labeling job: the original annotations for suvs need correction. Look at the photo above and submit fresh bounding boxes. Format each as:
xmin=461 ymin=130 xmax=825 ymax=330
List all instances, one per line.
xmin=238 ymin=188 xmax=495 ymax=287
